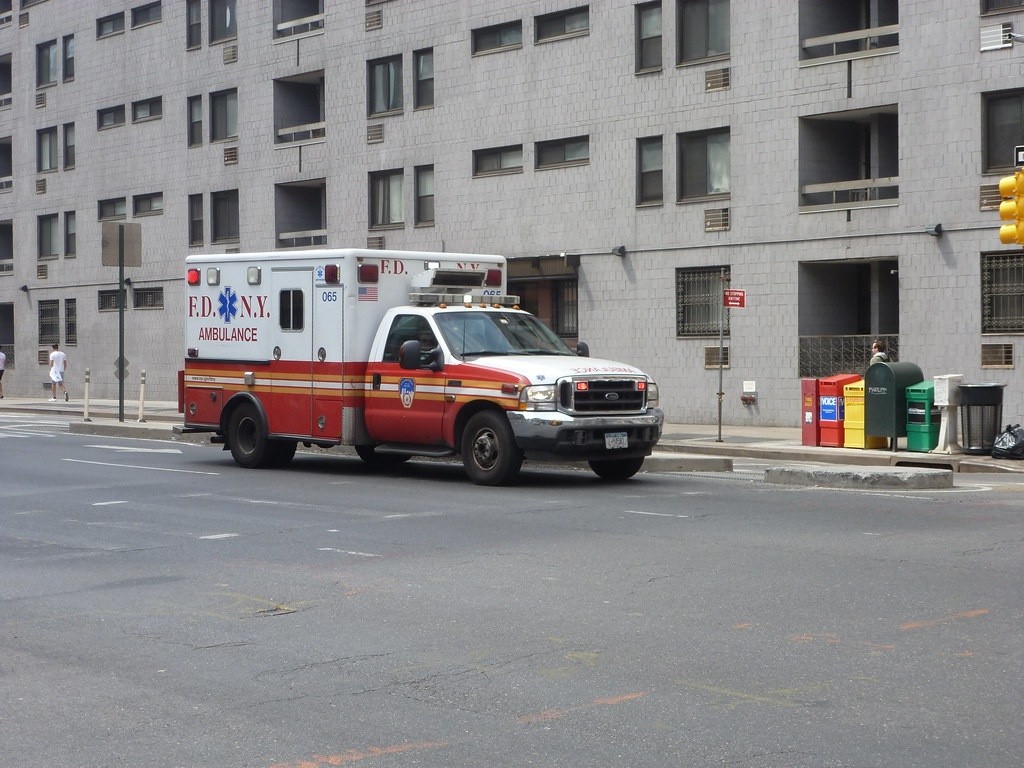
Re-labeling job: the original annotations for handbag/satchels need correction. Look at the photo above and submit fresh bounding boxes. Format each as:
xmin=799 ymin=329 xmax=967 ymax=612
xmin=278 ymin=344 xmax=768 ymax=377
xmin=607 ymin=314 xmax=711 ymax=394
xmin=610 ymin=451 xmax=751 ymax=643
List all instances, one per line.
xmin=50 ymin=367 xmax=62 ymax=382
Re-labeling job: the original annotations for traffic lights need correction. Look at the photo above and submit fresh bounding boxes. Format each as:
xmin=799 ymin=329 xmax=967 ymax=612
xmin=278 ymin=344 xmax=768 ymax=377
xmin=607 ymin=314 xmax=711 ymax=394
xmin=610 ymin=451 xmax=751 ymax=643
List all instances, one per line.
xmin=998 ymin=170 xmax=1024 ymax=246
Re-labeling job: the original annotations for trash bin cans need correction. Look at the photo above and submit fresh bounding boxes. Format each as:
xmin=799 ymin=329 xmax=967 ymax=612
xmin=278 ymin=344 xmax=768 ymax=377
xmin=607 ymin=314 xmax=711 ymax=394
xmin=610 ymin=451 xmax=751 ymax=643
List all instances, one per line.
xmin=906 ymin=380 xmax=941 ymax=452
xmin=818 ymin=373 xmax=861 ymax=447
xmin=957 ymin=380 xmax=1009 ymax=454
xmin=865 ymin=361 xmax=923 ymax=438
xmin=843 ymin=380 xmax=888 ymax=449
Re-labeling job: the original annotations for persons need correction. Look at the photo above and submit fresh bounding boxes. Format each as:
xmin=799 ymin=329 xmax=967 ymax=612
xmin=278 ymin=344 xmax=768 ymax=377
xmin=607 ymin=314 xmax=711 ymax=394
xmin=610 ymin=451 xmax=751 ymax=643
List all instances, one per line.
xmin=870 ymin=339 xmax=889 ymax=366
xmin=49 ymin=343 xmax=69 ymax=402
xmin=485 ymin=322 xmax=505 ymax=351
xmin=0 ymin=345 xmax=6 ymax=398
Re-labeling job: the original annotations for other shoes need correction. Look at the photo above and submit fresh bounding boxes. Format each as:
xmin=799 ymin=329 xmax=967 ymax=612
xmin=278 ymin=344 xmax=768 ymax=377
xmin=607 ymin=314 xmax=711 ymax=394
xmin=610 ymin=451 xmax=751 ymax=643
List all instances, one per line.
xmin=48 ymin=398 xmax=56 ymax=402
xmin=64 ymin=392 xmax=68 ymax=401
xmin=0 ymin=394 xmax=4 ymax=399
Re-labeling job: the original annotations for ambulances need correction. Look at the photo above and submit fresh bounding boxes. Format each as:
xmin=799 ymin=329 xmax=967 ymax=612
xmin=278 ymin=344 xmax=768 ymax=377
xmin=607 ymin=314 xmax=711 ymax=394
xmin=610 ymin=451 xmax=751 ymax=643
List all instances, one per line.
xmin=171 ymin=248 xmax=665 ymax=484
